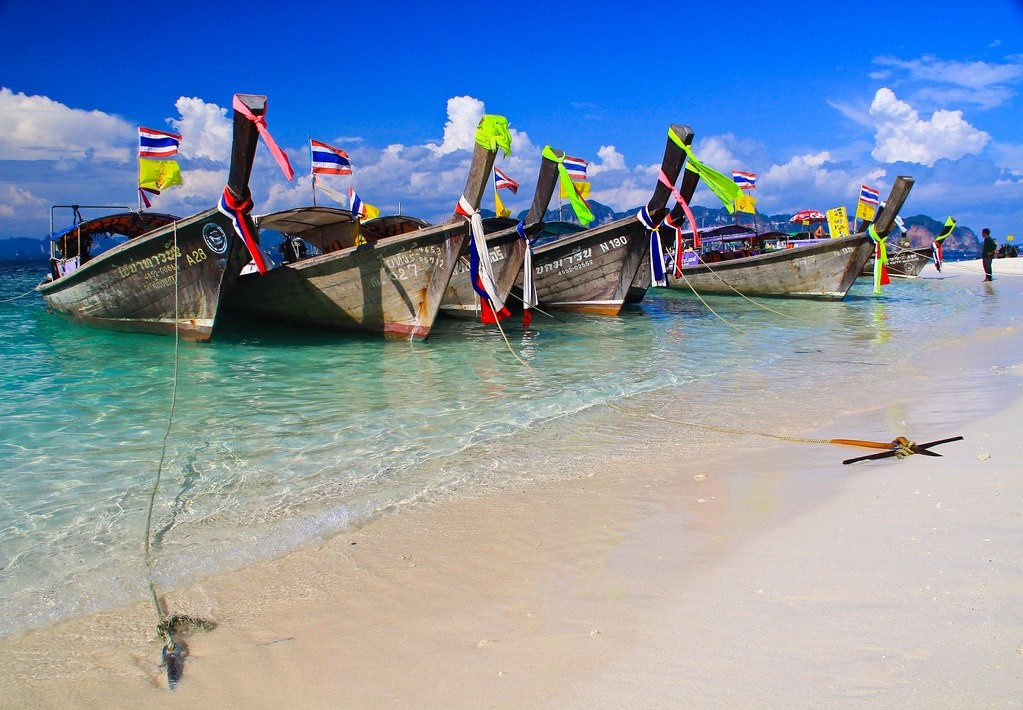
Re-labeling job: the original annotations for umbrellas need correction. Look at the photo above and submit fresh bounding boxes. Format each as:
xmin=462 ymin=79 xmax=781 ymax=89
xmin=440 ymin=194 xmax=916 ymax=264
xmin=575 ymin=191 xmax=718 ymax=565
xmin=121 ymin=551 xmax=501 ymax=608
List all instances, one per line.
xmin=789 ymin=208 xmax=827 ymax=239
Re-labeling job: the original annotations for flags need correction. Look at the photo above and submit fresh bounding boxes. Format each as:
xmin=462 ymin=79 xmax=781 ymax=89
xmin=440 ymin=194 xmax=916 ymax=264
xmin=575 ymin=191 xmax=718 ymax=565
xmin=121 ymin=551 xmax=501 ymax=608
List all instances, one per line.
xmin=734 ymin=193 xmax=757 ymax=214
xmin=139 ymin=127 xmax=182 ymax=157
xmin=139 ymin=158 xmax=183 ymax=191
xmin=860 ymin=184 xmax=880 ymax=204
xmin=495 ymin=166 xmax=519 ymax=195
xmin=732 ymin=169 xmax=756 ymax=190
xmin=560 ymin=181 xmax=592 ymax=199
xmin=560 ymin=154 xmax=589 ymax=181
xmin=495 ymin=194 xmax=512 ymax=217
xmin=858 ymin=204 xmax=877 ymax=222
xmin=311 ymin=139 xmax=352 ymax=175
xmin=349 ymin=187 xmax=380 ymax=223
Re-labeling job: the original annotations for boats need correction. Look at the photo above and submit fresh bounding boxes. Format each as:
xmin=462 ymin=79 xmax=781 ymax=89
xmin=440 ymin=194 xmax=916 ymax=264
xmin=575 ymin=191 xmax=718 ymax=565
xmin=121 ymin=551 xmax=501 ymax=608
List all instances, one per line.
xmin=858 ymin=216 xmax=957 ymax=280
xmin=30 ymin=88 xmax=269 ymax=346
xmin=362 ymin=146 xmax=567 ymax=327
xmin=225 ymin=114 xmax=515 ymax=344
xmin=483 ymin=124 xmax=694 ymax=319
xmin=662 ymin=174 xmax=916 ymax=302
xmin=548 ymin=160 xmax=701 ymax=309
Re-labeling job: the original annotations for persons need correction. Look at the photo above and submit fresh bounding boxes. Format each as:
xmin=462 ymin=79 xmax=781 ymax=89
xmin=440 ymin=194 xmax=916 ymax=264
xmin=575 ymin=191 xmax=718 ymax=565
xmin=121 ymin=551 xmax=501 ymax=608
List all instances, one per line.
xmin=982 ymin=228 xmax=998 ymax=282
xmin=814 ymin=225 xmax=825 ymax=239
